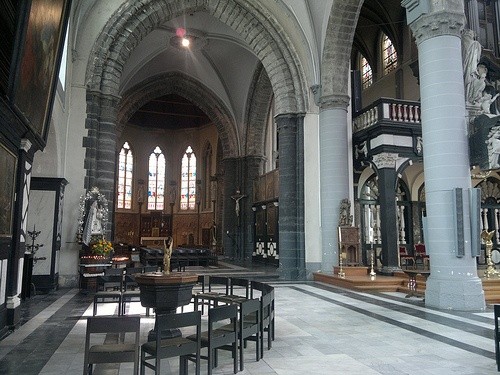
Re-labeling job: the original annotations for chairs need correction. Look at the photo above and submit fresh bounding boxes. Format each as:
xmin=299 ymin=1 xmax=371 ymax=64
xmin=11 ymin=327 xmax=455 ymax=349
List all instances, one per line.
xmin=84 ymin=274 xmax=274 ymax=375
xmin=414 ymin=243 xmax=430 ymax=270
xmin=399 ymin=247 xmax=417 ymax=270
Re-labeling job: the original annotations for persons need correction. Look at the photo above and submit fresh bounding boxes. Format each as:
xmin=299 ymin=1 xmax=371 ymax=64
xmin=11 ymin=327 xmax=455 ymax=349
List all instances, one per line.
xmin=461 ymin=29 xmax=482 ymax=105
xmin=488 ymin=126 xmax=500 ymax=169
xmin=163 ymin=239 xmax=173 ymax=274
xmin=169 ymin=185 xmax=175 ymax=202
xmin=232 ymin=195 xmax=243 ymax=216
xmin=196 ymin=185 xmax=202 ymax=199
xmin=137 ymin=186 xmax=144 ymax=202
xmin=482 ymin=230 xmax=499 ymax=277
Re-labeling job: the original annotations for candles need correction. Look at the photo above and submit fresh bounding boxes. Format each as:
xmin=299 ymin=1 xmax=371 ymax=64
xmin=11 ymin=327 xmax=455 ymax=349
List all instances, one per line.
xmin=339 ymin=227 xmax=342 ymax=241
